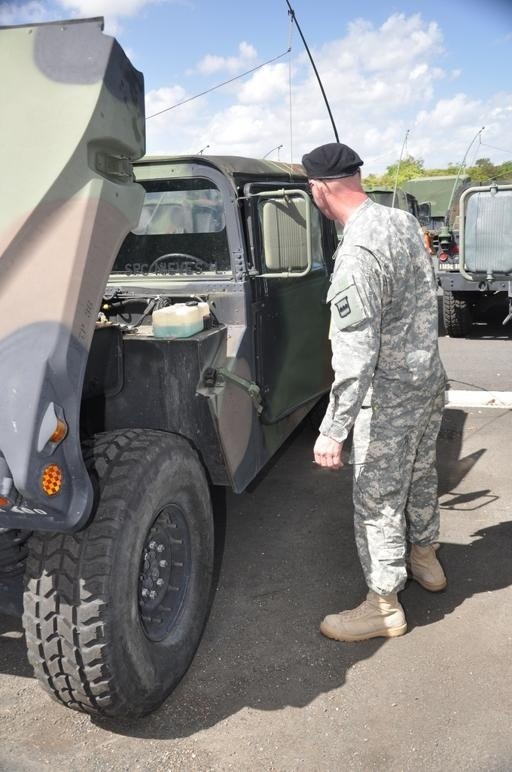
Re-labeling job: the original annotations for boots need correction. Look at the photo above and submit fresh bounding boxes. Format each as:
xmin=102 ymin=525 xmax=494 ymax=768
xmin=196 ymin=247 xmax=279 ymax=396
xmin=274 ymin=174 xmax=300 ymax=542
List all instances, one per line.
xmin=320 ymin=591 xmax=408 ymax=642
xmin=407 ymin=543 xmax=448 ymax=592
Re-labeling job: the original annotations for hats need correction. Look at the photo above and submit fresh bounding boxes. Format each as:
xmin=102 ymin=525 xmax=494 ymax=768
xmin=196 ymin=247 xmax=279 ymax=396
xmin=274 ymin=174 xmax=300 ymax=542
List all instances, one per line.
xmin=302 ymin=143 xmax=363 ymax=179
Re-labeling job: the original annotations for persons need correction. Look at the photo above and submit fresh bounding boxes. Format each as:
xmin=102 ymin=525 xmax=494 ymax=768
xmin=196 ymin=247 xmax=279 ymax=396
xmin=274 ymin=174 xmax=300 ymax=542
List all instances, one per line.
xmin=297 ymin=139 xmax=454 ymax=644
